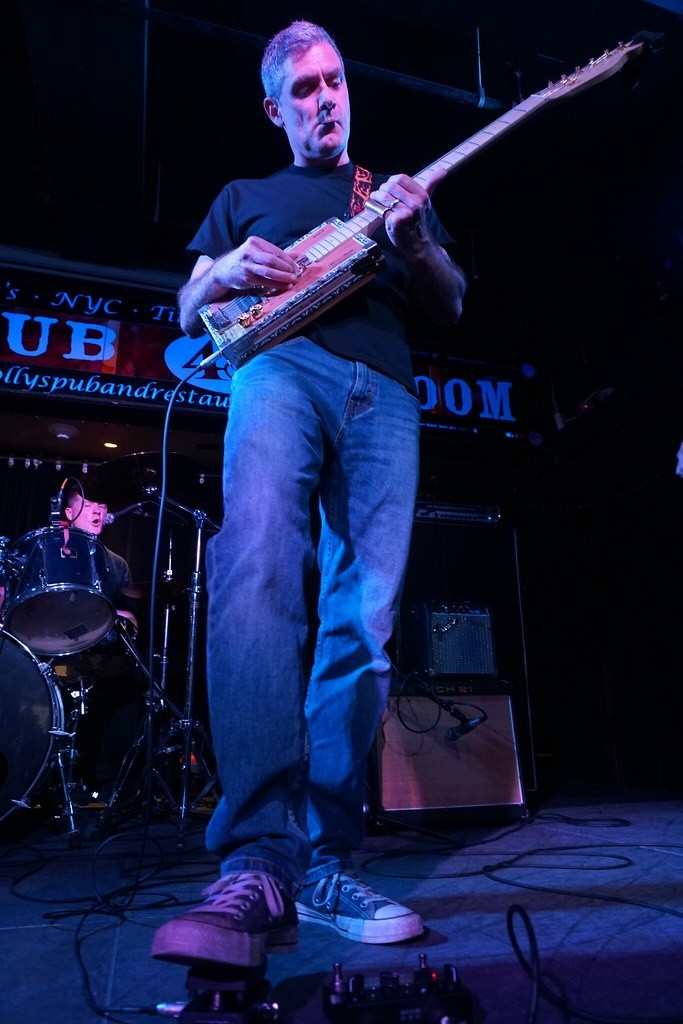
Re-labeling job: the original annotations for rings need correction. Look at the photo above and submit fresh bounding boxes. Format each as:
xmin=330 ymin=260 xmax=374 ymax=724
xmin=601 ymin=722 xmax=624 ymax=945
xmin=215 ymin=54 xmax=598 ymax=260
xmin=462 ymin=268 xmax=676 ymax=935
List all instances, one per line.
xmin=388 ymin=198 xmax=400 ymax=210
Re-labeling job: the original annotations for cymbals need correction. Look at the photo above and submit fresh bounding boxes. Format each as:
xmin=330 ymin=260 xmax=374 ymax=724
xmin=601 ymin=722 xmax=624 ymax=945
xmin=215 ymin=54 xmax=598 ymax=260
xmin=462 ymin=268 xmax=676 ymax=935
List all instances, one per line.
xmin=120 ymin=579 xmax=188 ymax=599
xmin=78 ymin=449 xmax=203 ymax=499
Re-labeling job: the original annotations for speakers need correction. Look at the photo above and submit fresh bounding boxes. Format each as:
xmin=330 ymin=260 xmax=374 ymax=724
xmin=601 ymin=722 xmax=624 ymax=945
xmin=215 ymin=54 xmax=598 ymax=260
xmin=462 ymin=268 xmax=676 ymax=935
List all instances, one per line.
xmin=370 ymin=676 xmax=532 ymax=836
xmin=407 ymin=600 xmax=498 ymax=681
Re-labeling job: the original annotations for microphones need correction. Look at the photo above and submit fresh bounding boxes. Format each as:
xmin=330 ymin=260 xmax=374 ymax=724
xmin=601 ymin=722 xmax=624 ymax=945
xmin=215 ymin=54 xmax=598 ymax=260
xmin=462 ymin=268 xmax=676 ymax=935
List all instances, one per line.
xmin=49 ymin=478 xmax=68 ymax=523
xmin=104 ymin=502 xmax=143 ymax=524
xmin=445 ymin=715 xmax=488 ymax=741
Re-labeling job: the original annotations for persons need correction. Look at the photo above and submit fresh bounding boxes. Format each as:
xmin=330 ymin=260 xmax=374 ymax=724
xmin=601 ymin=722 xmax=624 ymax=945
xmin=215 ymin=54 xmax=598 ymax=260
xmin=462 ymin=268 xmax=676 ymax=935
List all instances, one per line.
xmin=38 ymin=479 xmax=170 ymax=816
xmin=145 ymin=15 xmax=470 ymax=975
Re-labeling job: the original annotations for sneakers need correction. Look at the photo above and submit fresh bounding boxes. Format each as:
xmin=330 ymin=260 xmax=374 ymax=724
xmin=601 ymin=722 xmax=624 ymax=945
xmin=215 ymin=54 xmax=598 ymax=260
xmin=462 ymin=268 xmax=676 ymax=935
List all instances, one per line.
xmin=293 ymin=872 xmax=423 ymax=944
xmin=149 ymin=874 xmax=285 ymax=968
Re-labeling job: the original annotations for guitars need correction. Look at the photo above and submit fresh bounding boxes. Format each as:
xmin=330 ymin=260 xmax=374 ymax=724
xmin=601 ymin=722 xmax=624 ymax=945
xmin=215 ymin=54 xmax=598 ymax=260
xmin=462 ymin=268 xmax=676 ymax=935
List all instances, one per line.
xmin=197 ymin=38 xmax=646 ymax=373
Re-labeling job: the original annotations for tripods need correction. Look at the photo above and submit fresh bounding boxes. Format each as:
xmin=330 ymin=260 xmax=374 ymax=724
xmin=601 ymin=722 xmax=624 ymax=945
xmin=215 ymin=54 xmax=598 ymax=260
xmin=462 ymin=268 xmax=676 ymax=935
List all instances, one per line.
xmin=97 ymin=486 xmax=226 ymax=851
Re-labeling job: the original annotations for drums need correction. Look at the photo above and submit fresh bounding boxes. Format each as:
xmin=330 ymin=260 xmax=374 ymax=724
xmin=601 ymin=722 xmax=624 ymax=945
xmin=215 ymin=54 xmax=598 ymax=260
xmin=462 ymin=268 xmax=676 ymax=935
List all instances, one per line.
xmin=3 ymin=524 xmax=119 ymax=658
xmin=0 ymin=622 xmax=66 ymax=824
